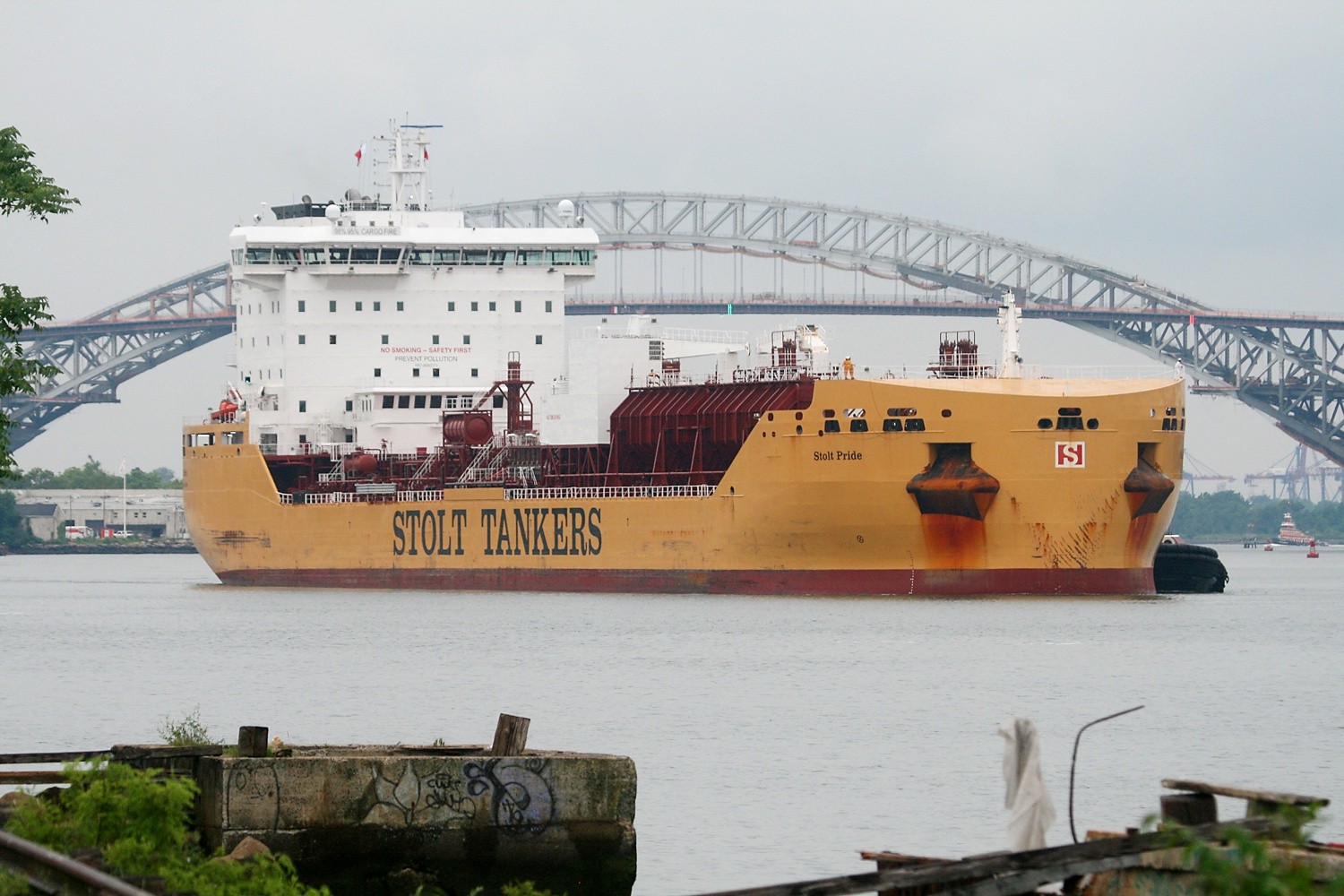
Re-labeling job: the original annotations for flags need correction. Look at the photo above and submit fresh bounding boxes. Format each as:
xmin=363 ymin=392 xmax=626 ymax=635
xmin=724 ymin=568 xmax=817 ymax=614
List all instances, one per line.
xmin=354 ymin=143 xmax=366 ymax=167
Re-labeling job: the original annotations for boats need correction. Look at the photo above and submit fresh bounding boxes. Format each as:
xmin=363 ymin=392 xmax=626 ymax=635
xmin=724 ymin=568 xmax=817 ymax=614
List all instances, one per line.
xmin=181 ymin=108 xmax=1186 ymax=597
xmin=1153 ymin=543 xmax=1230 ymax=593
xmin=1272 ymin=513 xmax=1314 ymax=545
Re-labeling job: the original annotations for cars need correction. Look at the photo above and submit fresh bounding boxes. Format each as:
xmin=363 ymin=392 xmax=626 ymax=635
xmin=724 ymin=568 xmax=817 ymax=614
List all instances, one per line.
xmin=754 ymin=292 xmax=781 ymax=300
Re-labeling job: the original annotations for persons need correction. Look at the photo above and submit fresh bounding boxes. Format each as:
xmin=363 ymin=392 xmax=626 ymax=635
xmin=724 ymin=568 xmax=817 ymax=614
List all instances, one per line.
xmin=843 ymin=357 xmax=855 ymax=380
xmin=1174 ymin=358 xmax=1183 ymax=380
